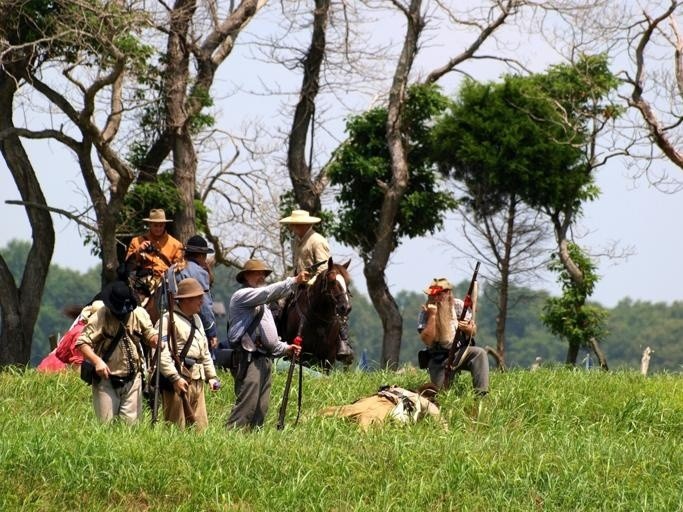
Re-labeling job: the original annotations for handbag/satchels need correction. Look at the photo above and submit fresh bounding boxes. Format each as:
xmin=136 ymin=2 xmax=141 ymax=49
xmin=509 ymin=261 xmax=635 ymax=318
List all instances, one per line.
xmin=219 ymin=349 xmax=239 ymax=369
xmin=418 ymin=348 xmax=429 ymax=370
xmin=55 ymin=319 xmax=88 ymax=363
xmin=150 ymin=359 xmax=183 ymax=392
xmin=81 ymin=360 xmax=94 ymax=385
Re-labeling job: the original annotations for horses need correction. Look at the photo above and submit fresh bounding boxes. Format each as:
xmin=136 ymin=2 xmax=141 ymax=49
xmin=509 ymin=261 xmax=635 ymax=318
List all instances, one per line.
xmin=277 ymin=257 xmax=353 ymax=376
xmin=64 ymin=251 xmax=162 ymax=327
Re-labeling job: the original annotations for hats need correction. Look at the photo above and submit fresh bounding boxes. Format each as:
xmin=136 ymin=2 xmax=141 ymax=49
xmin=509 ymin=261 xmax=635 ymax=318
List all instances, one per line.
xmin=178 ymin=236 xmax=214 ymax=254
xmin=236 ymin=260 xmax=273 ymax=283
xmin=423 ymin=278 xmax=452 ymax=295
xmin=142 ymin=208 xmax=174 ymax=223
xmin=279 ymin=209 xmax=321 ymax=224
xmin=102 ymin=281 xmax=138 ymax=315
xmin=173 ymin=278 xmax=205 ymax=299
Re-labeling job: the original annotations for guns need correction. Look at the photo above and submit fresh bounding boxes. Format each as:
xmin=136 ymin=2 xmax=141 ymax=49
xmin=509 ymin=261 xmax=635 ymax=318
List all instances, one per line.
xmin=278 ymin=315 xmax=306 ymax=430
xmin=443 ymin=262 xmax=481 ymax=392
xmin=151 ymin=276 xmax=165 ymax=423
xmin=304 ymin=260 xmax=328 ymax=276
xmin=164 ymin=267 xmax=197 ymax=428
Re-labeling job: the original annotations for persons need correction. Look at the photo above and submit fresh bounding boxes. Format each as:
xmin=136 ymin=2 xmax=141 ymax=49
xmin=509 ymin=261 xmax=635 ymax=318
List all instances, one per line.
xmin=280 ymin=210 xmax=353 ymax=361
xmin=224 ymin=259 xmax=314 ymax=429
xmin=416 ymin=276 xmax=492 ymax=398
xmin=74 ymin=280 xmax=167 ymax=427
xmin=149 ymin=277 xmax=220 ymax=434
xmin=123 ymin=208 xmax=186 ymax=308
xmin=154 ymin=234 xmax=219 ymax=363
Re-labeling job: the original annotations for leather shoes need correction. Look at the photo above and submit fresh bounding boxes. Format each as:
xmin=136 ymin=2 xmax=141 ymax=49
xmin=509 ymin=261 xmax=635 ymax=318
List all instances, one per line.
xmin=337 ymin=340 xmax=353 ymax=356
